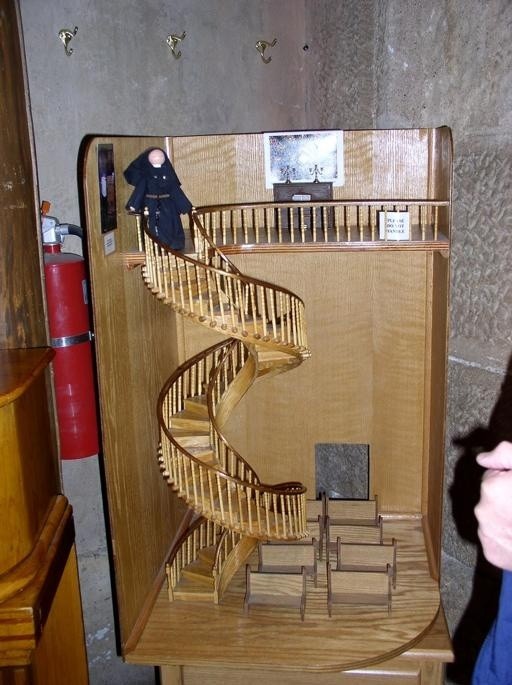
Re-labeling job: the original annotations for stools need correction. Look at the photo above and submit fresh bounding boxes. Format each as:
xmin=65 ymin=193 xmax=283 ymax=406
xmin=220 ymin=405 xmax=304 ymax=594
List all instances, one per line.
xmin=153 ymin=596 xmax=455 ymax=685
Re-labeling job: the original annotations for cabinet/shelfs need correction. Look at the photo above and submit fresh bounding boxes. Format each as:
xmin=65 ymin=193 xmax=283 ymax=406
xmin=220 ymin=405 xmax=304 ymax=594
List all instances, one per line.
xmin=0 ymin=344 xmax=89 ymax=681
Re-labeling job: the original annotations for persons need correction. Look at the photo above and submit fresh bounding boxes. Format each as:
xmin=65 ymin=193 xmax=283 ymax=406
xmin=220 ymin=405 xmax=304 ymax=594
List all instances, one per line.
xmin=469 ymin=440 xmax=512 ymax=685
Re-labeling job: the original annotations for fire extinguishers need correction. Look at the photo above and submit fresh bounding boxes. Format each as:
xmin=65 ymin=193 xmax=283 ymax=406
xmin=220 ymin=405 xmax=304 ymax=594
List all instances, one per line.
xmin=40 ymin=200 xmax=100 ymax=461
